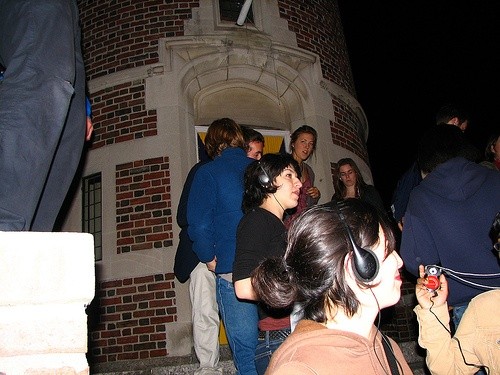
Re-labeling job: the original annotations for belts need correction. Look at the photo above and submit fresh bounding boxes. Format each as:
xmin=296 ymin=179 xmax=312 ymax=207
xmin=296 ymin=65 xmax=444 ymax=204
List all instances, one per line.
xmin=259 ymin=329 xmax=292 ymax=340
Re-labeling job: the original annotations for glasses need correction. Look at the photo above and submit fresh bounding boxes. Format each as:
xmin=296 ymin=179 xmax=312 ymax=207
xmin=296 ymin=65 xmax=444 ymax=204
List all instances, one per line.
xmin=494 ymin=242 xmax=500 ymax=252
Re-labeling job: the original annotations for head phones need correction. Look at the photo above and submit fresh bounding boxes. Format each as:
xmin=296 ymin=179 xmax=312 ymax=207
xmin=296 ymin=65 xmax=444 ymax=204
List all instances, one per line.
xmin=258 ymin=161 xmax=277 ymax=193
xmin=334 ymin=201 xmax=379 ymax=282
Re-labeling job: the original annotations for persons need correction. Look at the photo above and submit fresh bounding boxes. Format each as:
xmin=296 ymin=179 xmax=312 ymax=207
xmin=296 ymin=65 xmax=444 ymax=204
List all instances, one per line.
xmin=399 ymin=152 xmax=500 ymax=375
xmin=249 ymin=197 xmax=415 ymax=375
xmin=282 ymin=124 xmax=321 ymax=228
xmin=173 ymin=128 xmax=265 ymax=375
xmin=388 ymin=105 xmax=500 ymax=231
xmin=331 ymin=158 xmax=385 ymax=208
xmin=0 ymin=0 xmax=93 ymax=231
xmin=186 ymin=118 xmax=260 ymax=375
xmin=412 ymin=210 xmax=500 ymax=375
xmin=232 ymin=152 xmax=303 ymax=375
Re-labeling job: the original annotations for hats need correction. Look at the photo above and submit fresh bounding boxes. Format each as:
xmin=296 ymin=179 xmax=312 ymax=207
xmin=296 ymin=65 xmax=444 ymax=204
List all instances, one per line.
xmin=488 ymin=210 xmax=500 ymax=237
